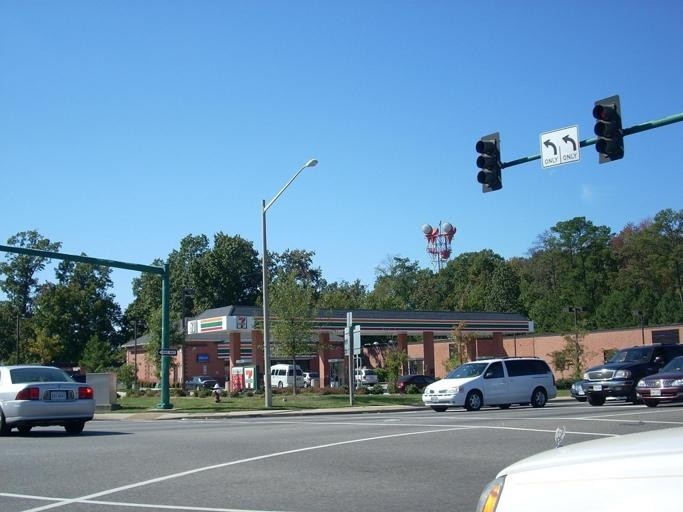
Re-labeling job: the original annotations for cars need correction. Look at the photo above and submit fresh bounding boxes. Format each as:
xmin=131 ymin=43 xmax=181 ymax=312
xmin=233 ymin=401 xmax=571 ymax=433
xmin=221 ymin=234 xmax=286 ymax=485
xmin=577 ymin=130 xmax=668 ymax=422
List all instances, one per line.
xmin=472 ymin=421 xmax=683 ymax=512
xmin=570 ymin=342 xmax=682 ymax=410
xmin=184 ymin=375 xmax=220 ymax=392
xmin=395 ymin=374 xmax=438 ymax=394
xmin=0 ymin=365 xmax=94 ymax=439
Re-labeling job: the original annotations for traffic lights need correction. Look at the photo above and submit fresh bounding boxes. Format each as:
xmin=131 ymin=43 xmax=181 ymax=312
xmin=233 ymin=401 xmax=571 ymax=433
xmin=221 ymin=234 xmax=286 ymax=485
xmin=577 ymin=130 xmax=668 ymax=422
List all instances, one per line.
xmin=592 ymin=95 xmax=626 ymax=165
xmin=475 ymin=131 xmax=503 ymax=193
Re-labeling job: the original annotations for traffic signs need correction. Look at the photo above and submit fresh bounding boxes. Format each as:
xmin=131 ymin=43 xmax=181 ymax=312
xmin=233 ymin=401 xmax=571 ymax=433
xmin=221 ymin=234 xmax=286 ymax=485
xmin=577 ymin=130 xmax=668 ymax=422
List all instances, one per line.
xmin=158 ymin=348 xmax=177 ymax=356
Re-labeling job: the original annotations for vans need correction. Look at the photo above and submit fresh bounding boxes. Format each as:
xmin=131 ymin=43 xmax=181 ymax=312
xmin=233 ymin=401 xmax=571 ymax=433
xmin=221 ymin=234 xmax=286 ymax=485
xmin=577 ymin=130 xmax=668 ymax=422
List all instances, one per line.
xmin=270 ymin=364 xmax=304 ymax=388
xmin=354 ymin=368 xmax=378 ymax=386
xmin=422 ymin=354 xmax=557 ymax=412
xmin=303 ymin=371 xmax=319 ymax=388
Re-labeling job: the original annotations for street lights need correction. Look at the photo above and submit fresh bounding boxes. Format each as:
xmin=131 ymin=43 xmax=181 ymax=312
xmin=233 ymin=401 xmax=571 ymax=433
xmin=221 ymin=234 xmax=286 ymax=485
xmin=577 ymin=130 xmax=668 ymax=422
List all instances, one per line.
xmin=14 ymin=311 xmax=33 ymax=364
xmin=128 ymin=314 xmax=145 ymax=388
xmin=258 ymin=156 xmax=317 ymax=407
